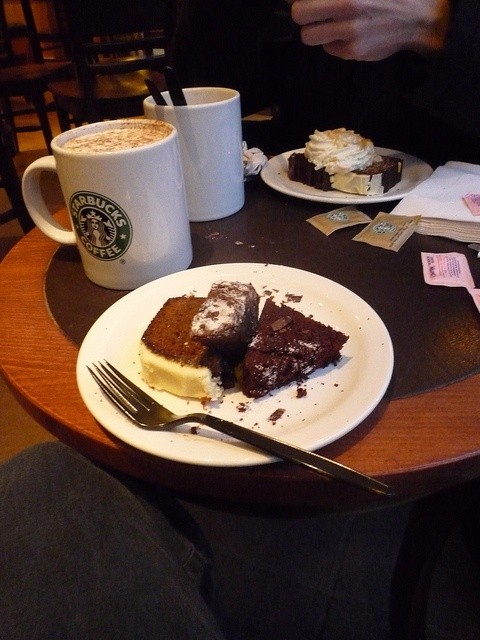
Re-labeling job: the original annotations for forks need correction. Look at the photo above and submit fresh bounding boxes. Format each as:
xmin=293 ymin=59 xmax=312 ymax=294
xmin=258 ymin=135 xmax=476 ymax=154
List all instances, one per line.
xmin=84 ymin=359 xmax=394 ymax=500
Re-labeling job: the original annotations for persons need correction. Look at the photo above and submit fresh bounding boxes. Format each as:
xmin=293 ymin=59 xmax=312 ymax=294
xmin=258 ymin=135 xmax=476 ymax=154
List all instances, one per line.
xmin=163 ymin=0 xmax=480 ymax=172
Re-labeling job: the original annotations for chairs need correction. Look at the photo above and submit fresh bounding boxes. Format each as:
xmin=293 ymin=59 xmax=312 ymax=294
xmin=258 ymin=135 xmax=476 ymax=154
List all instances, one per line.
xmin=0 ymin=0 xmax=77 ymax=157
xmin=44 ymin=1 xmax=168 ymax=160
xmin=0 ymin=108 xmax=67 ymax=236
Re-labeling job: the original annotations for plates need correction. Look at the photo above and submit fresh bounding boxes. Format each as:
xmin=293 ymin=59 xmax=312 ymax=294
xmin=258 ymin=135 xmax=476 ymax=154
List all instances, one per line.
xmin=74 ymin=261 xmax=396 ymax=468
xmin=261 ymin=146 xmax=434 ymax=206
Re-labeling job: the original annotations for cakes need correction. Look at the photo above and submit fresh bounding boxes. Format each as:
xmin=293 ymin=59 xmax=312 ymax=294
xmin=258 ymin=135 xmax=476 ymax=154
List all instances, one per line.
xmin=235 ymin=294 xmax=349 ymax=400
xmin=288 ymin=128 xmax=403 ymax=197
xmin=138 ymin=297 xmax=231 ymax=403
xmin=191 ymin=281 xmax=258 ymax=350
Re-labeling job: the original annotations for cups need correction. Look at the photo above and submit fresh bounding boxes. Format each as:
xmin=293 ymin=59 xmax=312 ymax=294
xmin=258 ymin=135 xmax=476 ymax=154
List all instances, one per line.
xmin=143 ymin=87 xmax=246 ymax=223
xmin=21 ymin=116 xmax=195 ymax=292
